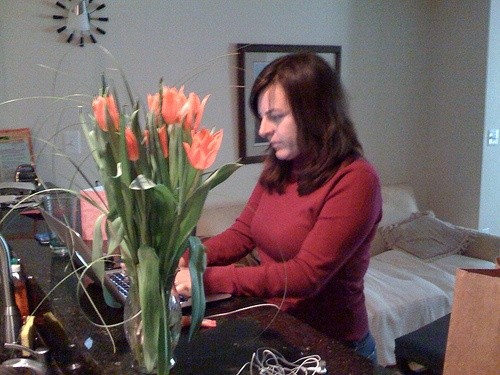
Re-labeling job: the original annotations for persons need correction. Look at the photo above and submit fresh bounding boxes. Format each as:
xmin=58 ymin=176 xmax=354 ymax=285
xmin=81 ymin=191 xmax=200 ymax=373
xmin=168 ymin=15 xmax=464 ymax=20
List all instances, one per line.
xmin=120 ymin=53 xmax=383 ymax=363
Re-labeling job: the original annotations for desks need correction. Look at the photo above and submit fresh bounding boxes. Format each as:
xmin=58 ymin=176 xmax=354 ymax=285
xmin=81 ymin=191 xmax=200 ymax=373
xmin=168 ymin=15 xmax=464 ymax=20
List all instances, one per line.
xmin=394 ymin=312 xmax=450 ymax=375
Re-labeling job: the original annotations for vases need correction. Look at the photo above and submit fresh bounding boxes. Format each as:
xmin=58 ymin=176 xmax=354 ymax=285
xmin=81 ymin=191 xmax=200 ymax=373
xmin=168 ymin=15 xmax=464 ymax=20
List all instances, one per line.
xmin=122 ymin=269 xmax=183 ymax=375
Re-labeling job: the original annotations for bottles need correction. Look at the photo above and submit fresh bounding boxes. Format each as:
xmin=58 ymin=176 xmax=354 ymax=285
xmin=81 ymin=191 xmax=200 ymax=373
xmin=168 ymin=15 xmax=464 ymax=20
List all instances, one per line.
xmin=11 ymin=264 xmax=29 ymax=324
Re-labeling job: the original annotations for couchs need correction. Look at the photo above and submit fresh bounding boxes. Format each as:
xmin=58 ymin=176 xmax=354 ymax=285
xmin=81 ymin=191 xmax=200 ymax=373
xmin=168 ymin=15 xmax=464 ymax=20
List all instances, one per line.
xmin=196 ymin=183 xmax=500 ymax=368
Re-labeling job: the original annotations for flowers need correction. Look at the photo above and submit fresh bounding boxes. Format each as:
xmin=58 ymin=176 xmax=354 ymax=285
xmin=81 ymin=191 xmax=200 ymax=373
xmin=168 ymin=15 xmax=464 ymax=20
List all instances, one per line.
xmin=0 ymin=42 xmax=345 ymax=357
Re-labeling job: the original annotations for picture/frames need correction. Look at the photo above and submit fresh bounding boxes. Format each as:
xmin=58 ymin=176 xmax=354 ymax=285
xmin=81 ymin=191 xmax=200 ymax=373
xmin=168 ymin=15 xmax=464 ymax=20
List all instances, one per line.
xmin=237 ymin=45 xmax=342 ymax=164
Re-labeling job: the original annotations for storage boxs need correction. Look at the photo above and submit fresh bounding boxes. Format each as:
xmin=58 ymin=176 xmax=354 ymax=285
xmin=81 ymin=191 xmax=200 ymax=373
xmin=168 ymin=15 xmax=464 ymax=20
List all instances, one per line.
xmin=79 ymin=185 xmax=111 ymax=241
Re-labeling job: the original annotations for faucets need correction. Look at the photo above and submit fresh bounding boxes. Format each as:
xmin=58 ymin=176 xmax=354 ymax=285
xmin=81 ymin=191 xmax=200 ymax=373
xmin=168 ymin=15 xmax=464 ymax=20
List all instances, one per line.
xmin=0 ymin=236 xmax=52 ymax=375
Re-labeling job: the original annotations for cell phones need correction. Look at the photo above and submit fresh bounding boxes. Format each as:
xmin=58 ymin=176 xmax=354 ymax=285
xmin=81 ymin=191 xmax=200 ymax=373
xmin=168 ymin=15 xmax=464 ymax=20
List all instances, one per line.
xmin=32 ymin=232 xmax=50 ymax=245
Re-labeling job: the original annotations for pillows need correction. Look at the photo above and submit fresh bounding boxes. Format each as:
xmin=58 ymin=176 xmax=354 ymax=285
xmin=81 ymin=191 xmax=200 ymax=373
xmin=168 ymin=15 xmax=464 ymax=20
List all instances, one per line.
xmin=377 ymin=209 xmax=471 ymax=260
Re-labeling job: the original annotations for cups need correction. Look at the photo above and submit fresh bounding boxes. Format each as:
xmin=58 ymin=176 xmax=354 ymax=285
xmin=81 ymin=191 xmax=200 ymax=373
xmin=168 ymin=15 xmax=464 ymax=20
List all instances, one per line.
xmin=33 ymin=214 xmax=54 ymax=244
xmin=43 ymin=193 xmax=76 ymax=253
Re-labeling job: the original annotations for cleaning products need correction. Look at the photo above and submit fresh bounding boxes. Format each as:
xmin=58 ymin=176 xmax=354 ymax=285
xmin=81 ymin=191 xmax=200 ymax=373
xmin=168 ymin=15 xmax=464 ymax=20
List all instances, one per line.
xmin=11 ymin=258 xmax=29 ymax=325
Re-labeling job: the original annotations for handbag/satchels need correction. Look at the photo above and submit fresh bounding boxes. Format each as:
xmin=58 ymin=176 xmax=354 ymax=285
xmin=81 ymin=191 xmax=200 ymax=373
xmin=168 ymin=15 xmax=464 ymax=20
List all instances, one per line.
xmin=81 ymin=186 xmax=109 ymax=241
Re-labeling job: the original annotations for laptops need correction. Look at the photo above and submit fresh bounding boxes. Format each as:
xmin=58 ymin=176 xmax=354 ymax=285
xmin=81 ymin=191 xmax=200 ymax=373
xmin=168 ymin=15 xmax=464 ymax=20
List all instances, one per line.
xmin=38 ymin=206 xmax=232 ymax=307
